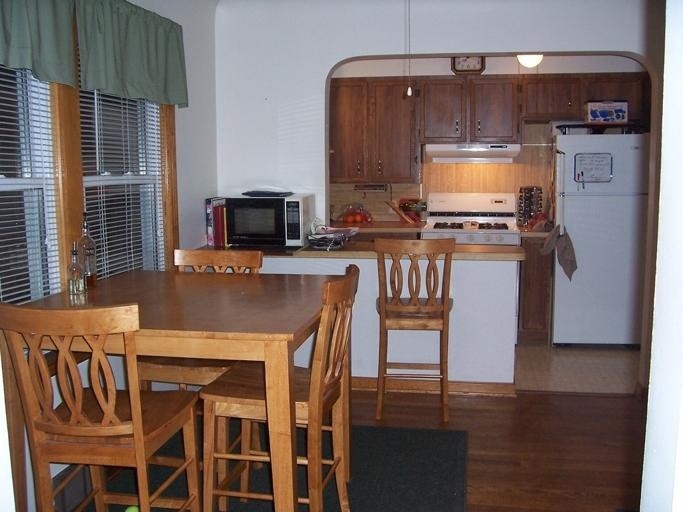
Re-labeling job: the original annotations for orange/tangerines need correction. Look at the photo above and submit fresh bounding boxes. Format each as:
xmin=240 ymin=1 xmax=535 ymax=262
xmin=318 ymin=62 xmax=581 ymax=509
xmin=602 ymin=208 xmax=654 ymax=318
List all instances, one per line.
xmin=125 ymin=505 xmax=139 ymax=512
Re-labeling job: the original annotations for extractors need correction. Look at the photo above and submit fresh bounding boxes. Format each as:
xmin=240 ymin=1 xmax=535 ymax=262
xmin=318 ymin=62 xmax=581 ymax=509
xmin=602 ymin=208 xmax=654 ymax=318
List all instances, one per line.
xmin=424 ymin=142 xmax=522 ymax=165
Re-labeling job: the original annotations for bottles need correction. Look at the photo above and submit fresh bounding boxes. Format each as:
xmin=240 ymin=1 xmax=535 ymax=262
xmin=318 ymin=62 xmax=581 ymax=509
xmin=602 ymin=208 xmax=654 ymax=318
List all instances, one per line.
xmin=66 ymin=210 xmax=97 ymax=297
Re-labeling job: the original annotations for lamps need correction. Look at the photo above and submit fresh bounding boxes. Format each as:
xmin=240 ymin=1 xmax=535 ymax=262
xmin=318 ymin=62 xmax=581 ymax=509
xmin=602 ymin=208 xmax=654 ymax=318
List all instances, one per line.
xmin=516 ymin=54 xmax=544 ymax=68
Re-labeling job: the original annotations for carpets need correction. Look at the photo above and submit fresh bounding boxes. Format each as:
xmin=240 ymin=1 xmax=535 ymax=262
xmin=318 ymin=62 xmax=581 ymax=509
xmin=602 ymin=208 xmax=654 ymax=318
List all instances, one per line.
xmin=52 ymin=415 xmax=469 ymax=512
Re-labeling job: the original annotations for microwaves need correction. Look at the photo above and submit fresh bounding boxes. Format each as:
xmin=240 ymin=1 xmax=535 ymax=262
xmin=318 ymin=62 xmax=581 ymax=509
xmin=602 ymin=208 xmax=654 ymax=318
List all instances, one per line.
xmin=224 ymin=192 xmax=316 ymax=249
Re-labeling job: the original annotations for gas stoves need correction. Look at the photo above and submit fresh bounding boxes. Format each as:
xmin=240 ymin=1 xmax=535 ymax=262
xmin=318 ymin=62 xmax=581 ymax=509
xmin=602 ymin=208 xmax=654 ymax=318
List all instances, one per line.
xmin=422 ymin=216 xmax=522 ymax=236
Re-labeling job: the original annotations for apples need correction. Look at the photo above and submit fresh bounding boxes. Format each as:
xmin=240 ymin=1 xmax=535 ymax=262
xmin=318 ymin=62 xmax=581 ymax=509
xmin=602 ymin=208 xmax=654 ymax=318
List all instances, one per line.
xmin=345 ymin=212 xmax=362 ymax=223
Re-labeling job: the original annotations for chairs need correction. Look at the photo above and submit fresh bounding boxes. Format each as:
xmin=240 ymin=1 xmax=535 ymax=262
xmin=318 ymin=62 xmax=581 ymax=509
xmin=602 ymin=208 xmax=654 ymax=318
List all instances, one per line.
xmin=0 ymin=299 xmax=200 ymax=511
xmin=138 ymin=248 xmax=263 ymax=511
xmin=374 ymin=237 xmax=454 ymax=421
xmin=199 ymin=263 xmax=360 ymax=512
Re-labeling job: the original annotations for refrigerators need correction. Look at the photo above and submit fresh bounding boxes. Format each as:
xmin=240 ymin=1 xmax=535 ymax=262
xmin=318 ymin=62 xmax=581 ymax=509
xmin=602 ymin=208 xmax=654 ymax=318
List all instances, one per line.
xmin=551 ymin=132 xmax=651 ymax=348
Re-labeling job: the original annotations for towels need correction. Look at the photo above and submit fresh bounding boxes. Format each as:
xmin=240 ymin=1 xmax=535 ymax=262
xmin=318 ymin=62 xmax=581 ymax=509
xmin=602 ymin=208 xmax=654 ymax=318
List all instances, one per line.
xmin=540 ymin=224 xmax=577 ymax=282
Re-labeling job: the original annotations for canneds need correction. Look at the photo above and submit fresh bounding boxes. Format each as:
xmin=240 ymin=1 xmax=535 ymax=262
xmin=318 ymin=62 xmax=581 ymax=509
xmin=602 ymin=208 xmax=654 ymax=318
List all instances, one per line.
xmin=419 ymin=210 xmax=430 ymax=221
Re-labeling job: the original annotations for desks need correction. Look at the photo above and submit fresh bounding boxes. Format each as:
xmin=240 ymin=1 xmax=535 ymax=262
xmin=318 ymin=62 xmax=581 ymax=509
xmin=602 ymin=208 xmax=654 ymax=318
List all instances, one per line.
xmin=0 ymin=268 xmax=344 ymax=512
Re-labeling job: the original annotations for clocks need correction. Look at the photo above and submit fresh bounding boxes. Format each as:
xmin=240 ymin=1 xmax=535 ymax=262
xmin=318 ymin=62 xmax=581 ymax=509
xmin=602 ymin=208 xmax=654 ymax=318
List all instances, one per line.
xmin=450 ymin=56 xmax=486 ymax=74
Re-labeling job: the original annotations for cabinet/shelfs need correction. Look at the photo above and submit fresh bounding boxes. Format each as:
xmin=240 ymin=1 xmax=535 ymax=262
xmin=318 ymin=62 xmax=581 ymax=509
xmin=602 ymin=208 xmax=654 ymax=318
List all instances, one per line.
xmin=521 ymin=73 xmax=644 ymax=122
xmin=330 ymin=77 xmax=417 ymax=184
xmin=518 ymin=237 xmax=552 ymax=346
xmin=419 ymin=77 xmax=519 ymax=145
